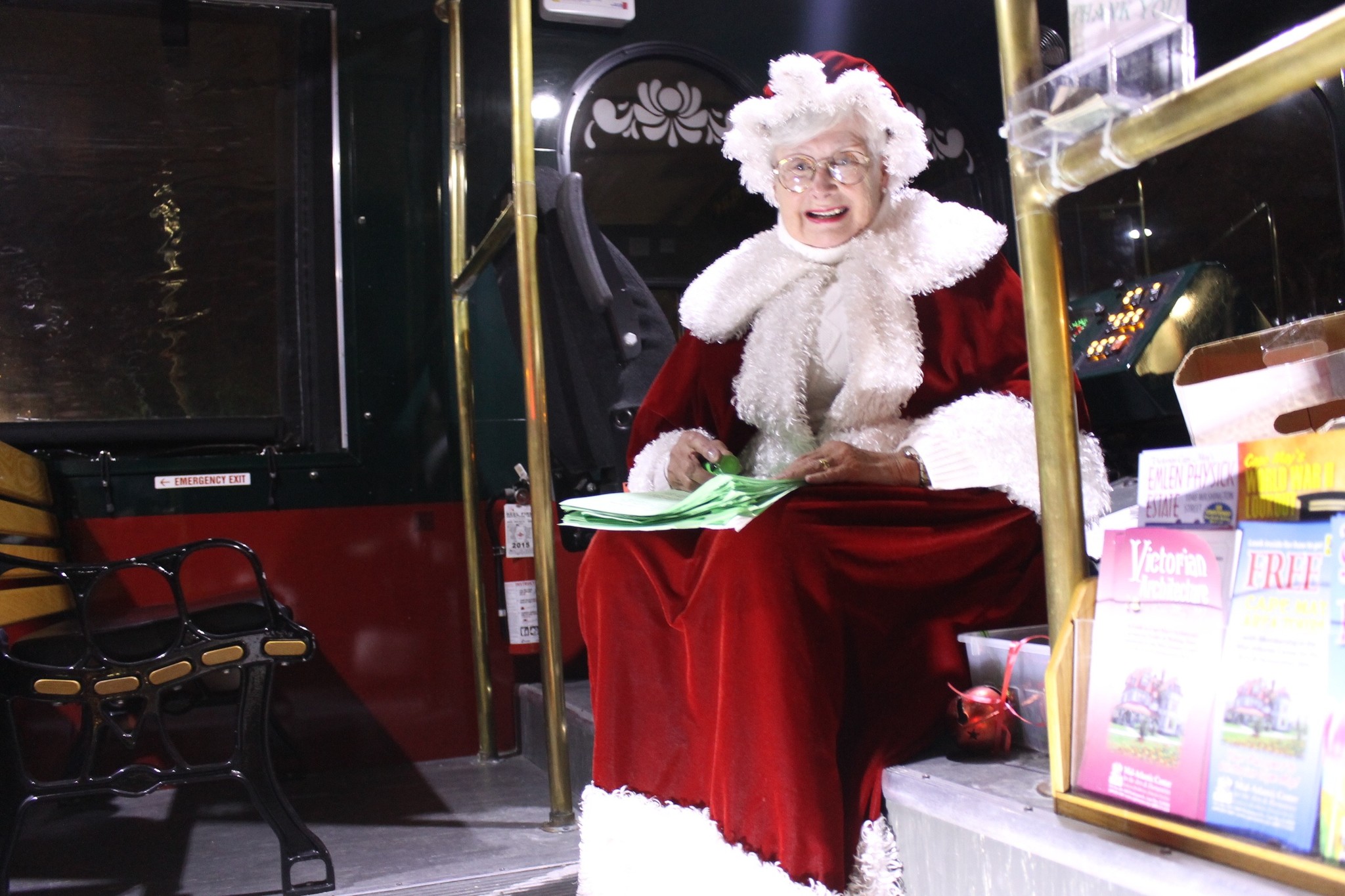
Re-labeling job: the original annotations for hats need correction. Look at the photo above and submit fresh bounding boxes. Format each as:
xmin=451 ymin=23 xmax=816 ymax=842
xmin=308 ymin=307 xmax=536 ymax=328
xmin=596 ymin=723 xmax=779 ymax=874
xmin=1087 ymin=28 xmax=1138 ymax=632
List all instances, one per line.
xmin=725 ymin=50 xmax=931 ymax=206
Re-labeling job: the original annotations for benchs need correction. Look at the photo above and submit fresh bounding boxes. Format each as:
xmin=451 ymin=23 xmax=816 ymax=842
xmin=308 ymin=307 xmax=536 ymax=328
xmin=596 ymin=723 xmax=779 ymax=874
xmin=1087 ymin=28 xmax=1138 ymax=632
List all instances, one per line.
xmin=0 ymin=441 xmax=335 ymax=895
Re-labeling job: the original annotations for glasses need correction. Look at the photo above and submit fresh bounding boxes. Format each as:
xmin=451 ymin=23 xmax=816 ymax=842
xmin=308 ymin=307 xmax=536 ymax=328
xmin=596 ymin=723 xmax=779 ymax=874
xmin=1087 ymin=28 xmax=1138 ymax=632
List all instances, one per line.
xmin=772 ymin=152 xmax=877 ymax=194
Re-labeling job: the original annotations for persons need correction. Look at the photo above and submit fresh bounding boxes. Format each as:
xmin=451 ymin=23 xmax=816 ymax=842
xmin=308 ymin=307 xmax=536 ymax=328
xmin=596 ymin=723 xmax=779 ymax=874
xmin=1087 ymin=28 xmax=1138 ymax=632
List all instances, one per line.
xmin=575 ymin=47 xmax=1108 ymax=895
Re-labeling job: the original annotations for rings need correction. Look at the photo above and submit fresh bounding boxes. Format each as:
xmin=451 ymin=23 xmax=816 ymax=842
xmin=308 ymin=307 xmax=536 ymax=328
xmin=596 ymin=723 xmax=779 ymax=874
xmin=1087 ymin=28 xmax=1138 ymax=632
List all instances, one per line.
xmin=819 ymin=459 xmax=831 ymax=471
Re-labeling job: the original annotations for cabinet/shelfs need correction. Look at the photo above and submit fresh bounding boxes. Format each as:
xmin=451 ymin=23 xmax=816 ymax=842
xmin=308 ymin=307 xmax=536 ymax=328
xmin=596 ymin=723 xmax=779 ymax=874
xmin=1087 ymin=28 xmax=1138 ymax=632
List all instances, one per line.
xmin=999 ymin=4 xmax=1345 ymax=896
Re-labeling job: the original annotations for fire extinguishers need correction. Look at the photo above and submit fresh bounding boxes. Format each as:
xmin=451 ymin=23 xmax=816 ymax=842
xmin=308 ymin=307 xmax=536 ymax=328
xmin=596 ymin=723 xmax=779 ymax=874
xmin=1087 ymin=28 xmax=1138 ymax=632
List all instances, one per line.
xmin=482 ymin=460 xmax=544 ymax=655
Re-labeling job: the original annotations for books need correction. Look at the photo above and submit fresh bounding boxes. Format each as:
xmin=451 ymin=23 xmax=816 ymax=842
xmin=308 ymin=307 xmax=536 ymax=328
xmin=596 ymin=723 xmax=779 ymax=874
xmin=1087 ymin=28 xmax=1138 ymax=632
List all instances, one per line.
xmin=1077 ymin=436 xmax=1345 ymax=869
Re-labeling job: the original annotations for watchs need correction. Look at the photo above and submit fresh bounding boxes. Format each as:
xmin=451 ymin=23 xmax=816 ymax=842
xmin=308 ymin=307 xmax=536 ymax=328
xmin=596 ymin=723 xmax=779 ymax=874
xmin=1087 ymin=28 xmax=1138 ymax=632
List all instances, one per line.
xmin=904 ymin=445 xmax=930 ymax=487
